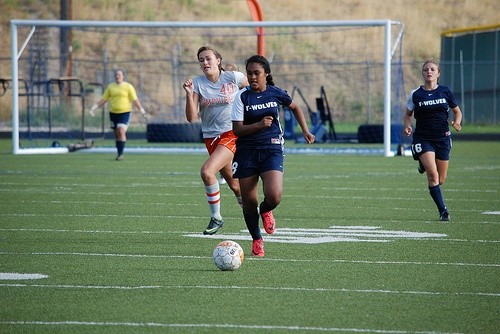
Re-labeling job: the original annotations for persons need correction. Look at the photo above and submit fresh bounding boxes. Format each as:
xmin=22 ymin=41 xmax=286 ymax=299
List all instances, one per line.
xmin=404 ymin=58 xmax=465 ymax=220
xmin=186 ymin=50 xmax=260 ymax=235
xmin=230 ymin=54 xmax=316 ymax=258
xmin=90 ymin=69 xmax=147 ymax=161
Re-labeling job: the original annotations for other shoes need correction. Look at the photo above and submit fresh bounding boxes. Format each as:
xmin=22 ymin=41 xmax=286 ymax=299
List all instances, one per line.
xmin=116 ymin=155 xmax=123 ymax=160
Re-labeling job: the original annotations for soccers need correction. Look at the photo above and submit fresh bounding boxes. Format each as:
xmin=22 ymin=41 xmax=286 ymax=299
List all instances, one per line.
xmin=213 ymin=240 xmax=244 ymax=270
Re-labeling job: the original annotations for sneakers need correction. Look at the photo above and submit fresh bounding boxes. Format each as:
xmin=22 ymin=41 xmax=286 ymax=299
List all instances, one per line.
xmin=203 ymin=216 xmax=224 ymax=235
xmin=252 ymin=236 xmax=264 ymax=257
xmin=440 ymin=211 xmax=450 ymax=221
xmin=418 ymin=160 xmax=426 ymax=174
xmin=260 ymin=211 xmax=275 ymax=234
